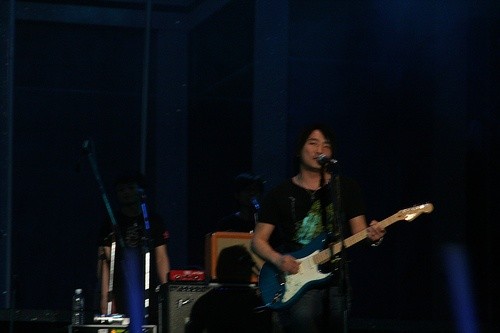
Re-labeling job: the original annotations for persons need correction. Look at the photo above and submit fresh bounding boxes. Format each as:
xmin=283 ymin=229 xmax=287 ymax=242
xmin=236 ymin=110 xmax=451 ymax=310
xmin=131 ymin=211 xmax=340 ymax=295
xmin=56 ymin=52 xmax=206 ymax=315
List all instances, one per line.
xmin=220 ymin=178 xmax=264 ymax=234
xmin=96 ymin=172 xmax=174 ymax=316
xmin=251 ymin=120 xmax=388 ymax=333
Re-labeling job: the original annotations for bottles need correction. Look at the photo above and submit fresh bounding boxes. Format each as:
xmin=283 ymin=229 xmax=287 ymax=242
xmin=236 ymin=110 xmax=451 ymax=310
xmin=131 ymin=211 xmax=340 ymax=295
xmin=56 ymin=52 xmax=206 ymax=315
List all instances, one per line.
xmin=73 ymin=289 xmax=84 ymax=325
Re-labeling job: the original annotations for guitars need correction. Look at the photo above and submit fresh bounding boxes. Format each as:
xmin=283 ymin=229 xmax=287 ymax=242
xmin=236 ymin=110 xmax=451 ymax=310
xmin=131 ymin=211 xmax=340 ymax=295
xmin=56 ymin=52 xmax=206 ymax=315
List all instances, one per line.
xmin=257 ymin=202 xmax=434 ymax=313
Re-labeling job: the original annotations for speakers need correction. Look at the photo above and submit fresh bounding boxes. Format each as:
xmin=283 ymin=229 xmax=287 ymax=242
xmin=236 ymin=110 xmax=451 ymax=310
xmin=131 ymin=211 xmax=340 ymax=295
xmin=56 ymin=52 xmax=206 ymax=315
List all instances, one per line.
xmin=159 ymin=232 xmax=271 ymax=333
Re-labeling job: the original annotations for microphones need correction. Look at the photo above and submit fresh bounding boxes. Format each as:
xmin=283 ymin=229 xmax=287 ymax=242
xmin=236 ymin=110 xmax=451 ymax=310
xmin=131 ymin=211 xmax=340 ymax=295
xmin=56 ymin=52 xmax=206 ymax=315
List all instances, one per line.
xmin=317 ymin=154 xmax=344 ymax=166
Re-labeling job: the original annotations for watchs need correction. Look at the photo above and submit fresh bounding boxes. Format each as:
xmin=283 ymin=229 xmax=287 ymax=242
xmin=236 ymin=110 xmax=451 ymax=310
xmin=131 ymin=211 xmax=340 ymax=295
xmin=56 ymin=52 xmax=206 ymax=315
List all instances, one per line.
xmin=370 ymin=238 xmax=384 ymax=247
